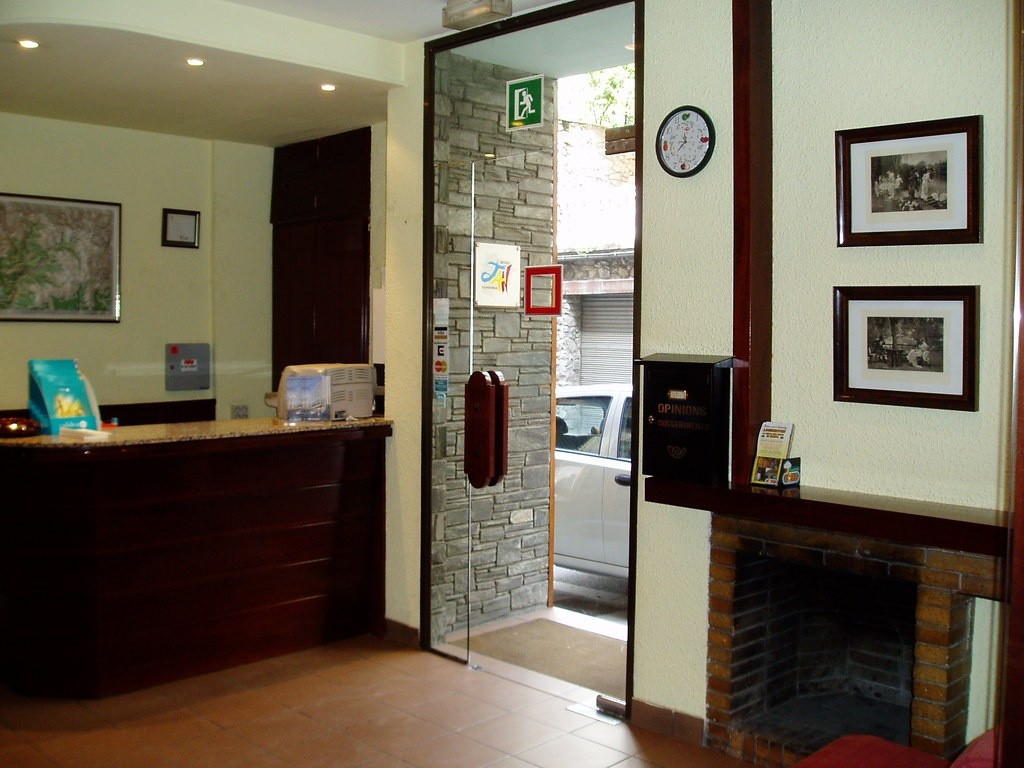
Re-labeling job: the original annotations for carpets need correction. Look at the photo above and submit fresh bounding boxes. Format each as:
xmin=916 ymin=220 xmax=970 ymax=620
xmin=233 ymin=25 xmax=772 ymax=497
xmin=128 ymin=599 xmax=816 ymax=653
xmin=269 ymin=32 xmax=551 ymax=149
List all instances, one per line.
xmin=448 ymin=617 xmax=627 ymax=702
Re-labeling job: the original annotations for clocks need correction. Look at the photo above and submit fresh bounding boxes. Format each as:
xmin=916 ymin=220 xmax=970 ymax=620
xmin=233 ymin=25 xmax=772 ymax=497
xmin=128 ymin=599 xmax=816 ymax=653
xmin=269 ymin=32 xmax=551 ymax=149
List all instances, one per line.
xmin=655 ymin=106 xmax=715 ymax=177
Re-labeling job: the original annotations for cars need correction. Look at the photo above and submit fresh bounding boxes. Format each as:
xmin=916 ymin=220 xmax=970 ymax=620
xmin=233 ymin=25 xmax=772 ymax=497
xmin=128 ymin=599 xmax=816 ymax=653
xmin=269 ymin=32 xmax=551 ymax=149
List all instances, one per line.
xmin=554 ymin=383 xmax=634 ymax=578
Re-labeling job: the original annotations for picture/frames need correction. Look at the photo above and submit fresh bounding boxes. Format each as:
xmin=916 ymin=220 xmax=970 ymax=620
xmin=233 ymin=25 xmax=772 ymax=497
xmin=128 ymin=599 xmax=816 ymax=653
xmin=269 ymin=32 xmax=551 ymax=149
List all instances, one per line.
xmin=836 ymin=114 xmax=985 ymax=249
xmin=833 ymin=284 xmax=980 ymax=413
xmin=1 ymin=192 xmax=122 ymax=326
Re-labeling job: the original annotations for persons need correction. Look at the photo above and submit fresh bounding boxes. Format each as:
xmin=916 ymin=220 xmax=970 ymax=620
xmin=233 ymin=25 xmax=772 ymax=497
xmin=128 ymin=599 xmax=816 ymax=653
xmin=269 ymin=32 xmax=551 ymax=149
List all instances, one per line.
xmin=908 ymin=167 xmax=934 ymax=201
xmin=908 ymin=338 xmax=929 ymax=368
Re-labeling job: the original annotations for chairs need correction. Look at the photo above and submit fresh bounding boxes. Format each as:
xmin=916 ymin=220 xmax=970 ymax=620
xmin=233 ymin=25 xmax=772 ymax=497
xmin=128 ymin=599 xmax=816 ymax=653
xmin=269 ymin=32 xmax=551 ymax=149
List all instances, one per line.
xmin=790 ymin=728 xmax=995 ymax=768
